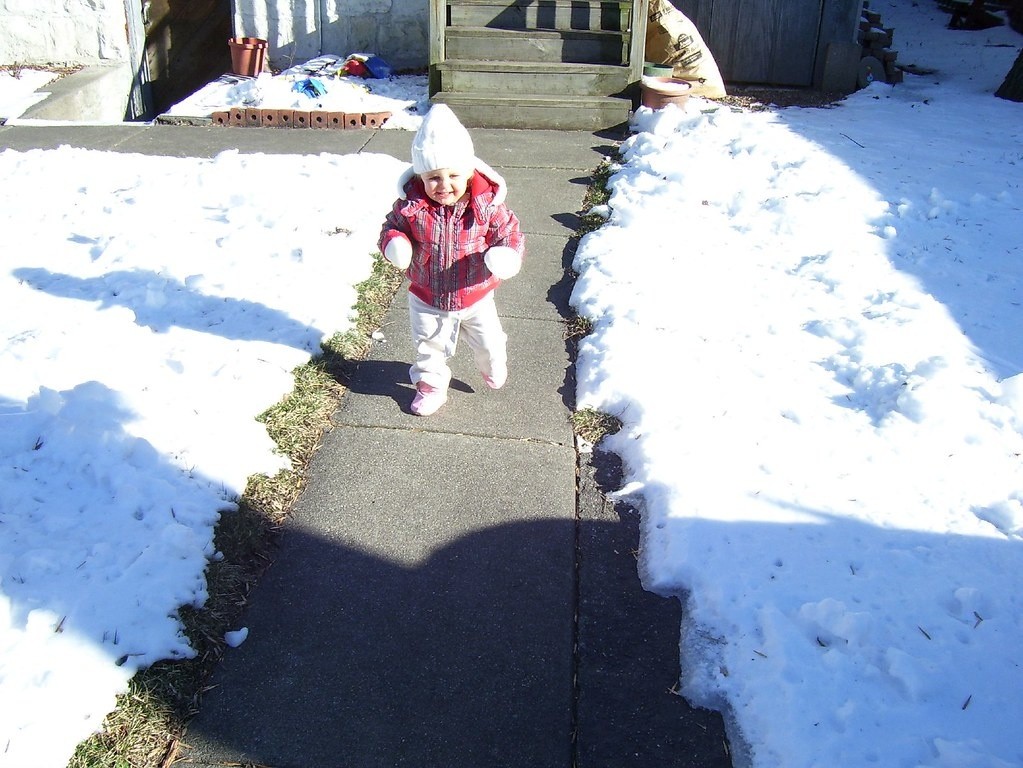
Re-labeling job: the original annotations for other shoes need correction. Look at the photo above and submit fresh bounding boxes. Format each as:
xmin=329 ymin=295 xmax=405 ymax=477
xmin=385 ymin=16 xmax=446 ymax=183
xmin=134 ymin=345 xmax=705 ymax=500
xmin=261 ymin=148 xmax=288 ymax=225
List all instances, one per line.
xmin=480 ymin=371 xmax=508 ymax=390
xmin=410 ymin=381 xmax=447 ymax=417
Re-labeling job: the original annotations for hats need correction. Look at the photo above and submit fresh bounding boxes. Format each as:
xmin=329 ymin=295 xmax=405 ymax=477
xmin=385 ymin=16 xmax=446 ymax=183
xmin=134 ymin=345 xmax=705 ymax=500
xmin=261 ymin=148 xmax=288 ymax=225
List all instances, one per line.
xmin=412 ymin=104 xmax=475 ymax=174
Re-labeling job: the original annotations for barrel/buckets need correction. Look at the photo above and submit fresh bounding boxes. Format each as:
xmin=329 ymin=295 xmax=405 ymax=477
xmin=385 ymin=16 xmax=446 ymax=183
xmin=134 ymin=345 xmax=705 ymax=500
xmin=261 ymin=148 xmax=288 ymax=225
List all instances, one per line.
xmin=228 ymin=37 xmax=268 ymax=75
xmin=643 ymin=64 xmax=674 ymax=78
xmin=640 ymin=77 xmax=692 ymax=110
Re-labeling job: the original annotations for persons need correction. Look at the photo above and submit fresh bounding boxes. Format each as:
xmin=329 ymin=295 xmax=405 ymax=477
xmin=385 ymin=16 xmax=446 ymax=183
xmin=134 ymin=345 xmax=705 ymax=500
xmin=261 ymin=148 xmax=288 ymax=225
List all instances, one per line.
xmin=377 ymin=104 xmax=525 ymax=416
